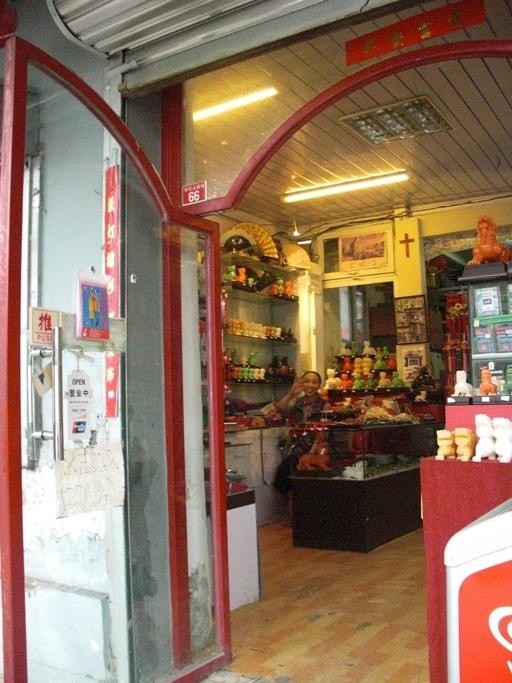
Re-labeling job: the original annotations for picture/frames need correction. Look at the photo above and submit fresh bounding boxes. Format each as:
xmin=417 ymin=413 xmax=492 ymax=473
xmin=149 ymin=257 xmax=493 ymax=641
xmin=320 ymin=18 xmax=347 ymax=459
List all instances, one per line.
xmin=394 ymin=295 xmax=427 ymax=344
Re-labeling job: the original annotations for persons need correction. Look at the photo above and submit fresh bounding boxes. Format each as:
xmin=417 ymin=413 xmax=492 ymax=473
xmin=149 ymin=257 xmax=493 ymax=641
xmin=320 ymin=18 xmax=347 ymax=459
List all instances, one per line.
xmin=277 ymin=371 xmax=325 ymax=529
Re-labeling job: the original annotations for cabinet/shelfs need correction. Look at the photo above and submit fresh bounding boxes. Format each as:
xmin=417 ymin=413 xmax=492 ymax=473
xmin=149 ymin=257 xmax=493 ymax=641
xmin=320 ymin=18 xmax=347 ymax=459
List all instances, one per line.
xmin=222 ymin=254 xmax=299 ymax=528
xmin=460 ymin=263 xmax=511 ymax=403
